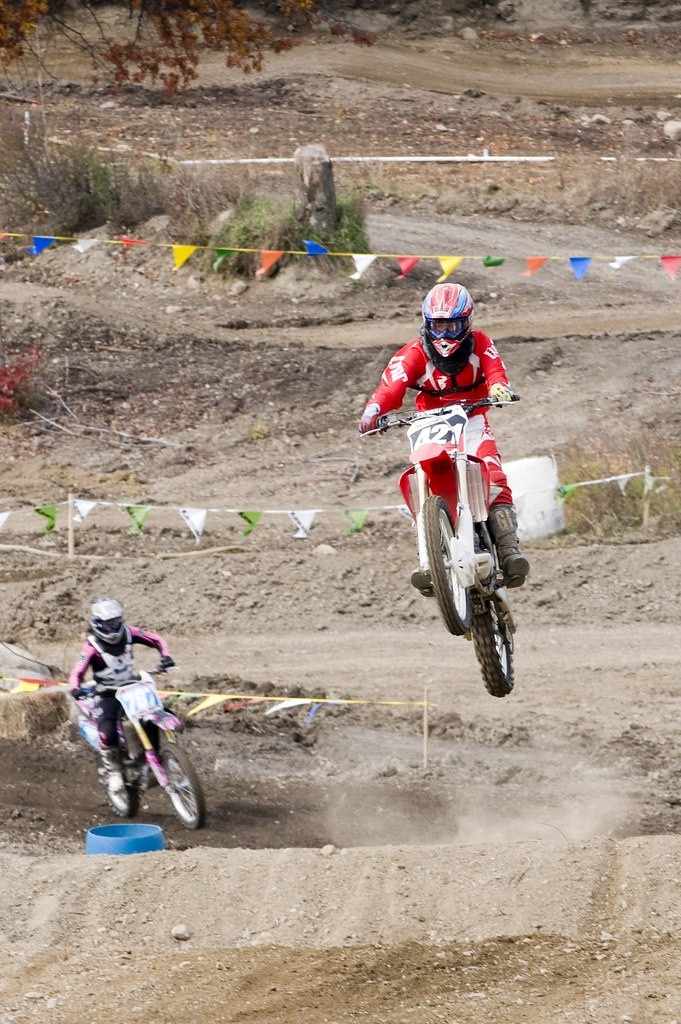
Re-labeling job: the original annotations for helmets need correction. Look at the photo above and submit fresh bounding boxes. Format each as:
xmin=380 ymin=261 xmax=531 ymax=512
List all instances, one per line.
xmin=90 ymin=599 xmax=126 ymax=644
xmin=421 ymin=283 xmax=474 ymax=358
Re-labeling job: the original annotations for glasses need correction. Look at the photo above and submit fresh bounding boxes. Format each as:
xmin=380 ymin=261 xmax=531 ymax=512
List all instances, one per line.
xmin=101 ymin=617 xmax=121 ymax=634
xmin=425 ymin=315 xmax=468 ymax=339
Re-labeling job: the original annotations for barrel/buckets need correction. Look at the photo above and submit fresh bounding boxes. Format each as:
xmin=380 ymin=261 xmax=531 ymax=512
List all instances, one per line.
xmin=500 ymin=456 xmax=568 ymax=542
xmin=85 ymin=821 xmax=166 ymax=855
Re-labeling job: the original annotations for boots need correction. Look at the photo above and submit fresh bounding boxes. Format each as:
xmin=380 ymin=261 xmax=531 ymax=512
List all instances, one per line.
xmin=489 ymin=505 xmax=530 ymax=588
xmin=411 ymin=522 xmax=436 ymax=597
xmin=101 ymin=748 xmax=131 ymax=817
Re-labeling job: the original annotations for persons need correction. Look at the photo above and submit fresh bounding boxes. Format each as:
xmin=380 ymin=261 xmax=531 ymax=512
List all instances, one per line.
xmin=359 ymin=283 xmax=529 ymax=596
xmin=71 ymin=600 xmax=173 ymax=792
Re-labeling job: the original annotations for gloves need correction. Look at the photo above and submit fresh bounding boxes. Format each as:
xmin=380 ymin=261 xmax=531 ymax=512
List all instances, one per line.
xmin=487 ymin=382 xmax=514 ymax=408
xmin=161 ymin=656 xmax=175 ymax=668
xmin=71 ymin=688 xmax=88 ymax=700
xmin=358 ymin=402 xmax=385 ymax=436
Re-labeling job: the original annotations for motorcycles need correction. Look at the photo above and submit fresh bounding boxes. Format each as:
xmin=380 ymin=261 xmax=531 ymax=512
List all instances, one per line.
xmin=68 ymin=660 xmax=208 ymax=832
xmin=357 ymin=395 xmax=522 ymax=699
